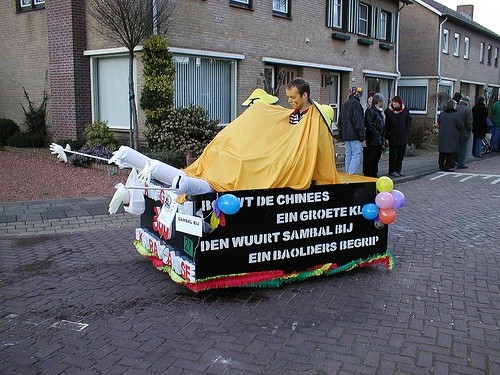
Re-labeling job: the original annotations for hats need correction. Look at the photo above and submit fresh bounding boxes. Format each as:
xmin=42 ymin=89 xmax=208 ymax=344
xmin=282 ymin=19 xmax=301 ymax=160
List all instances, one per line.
xmin=311 ymin=98 xmax=334 ymax=136
xmin=241 ymin=88 xmax=279 ymax=108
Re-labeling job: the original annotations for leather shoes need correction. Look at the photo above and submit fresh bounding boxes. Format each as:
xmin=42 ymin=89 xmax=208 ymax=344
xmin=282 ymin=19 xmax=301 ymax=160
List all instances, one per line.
xmin=398 ymin=172 xmax=405 ymax=176
xmin=388 ymin=171 xmax=400 ymax=177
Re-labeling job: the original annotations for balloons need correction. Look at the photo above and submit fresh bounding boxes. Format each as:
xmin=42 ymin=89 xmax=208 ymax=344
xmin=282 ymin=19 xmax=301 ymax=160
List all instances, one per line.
xmin=211 ymin=193 xmax=241 ymax=230
xmin=361 ymin=176 xmax=405 ymax=224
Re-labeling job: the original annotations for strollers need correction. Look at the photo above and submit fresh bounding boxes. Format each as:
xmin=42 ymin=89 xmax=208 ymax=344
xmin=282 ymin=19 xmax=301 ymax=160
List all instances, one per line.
xmin=479 ymin=117 xmax=494 ymax=155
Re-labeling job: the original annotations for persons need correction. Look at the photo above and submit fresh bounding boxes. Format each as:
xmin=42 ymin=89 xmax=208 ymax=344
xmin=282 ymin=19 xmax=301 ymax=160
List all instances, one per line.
xmin=437 ymin=93 xmax=500 ymax=172
xmin=285 ymin=78 xmax=312 ymax=125
xmin=337 ymin=85 xmax=412 ymax=179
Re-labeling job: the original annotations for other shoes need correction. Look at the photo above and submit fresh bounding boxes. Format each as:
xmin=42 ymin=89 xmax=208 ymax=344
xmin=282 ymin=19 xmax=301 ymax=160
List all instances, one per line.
xmin=439 ymin=168 xmax=456 ymax=171
xmin=457 ymin=165 xmax=468 ymax=169
xmin=473 ymin=154 xmax=482 ymax=157
